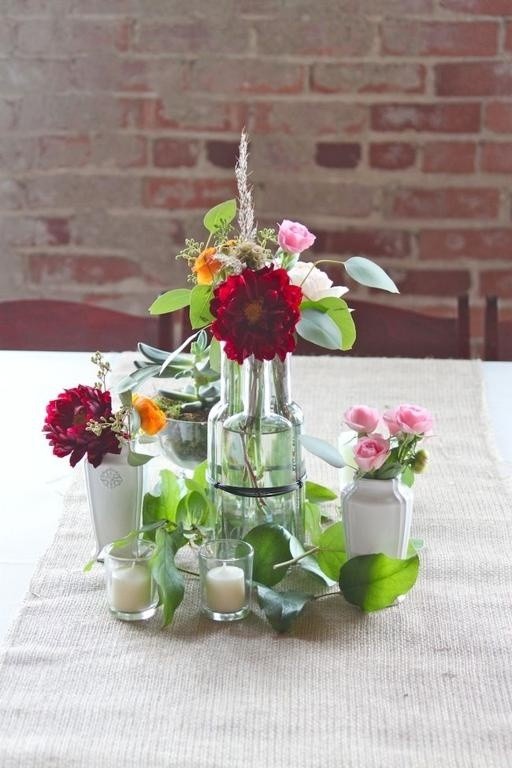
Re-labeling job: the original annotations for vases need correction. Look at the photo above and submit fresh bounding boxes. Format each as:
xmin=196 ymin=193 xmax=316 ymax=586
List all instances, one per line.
xmin=85 ymin=442 xmax=143 ymax=563
xmin=208 ymin=338 xmax=305 ymax=590
xmin=341 ymin=478 xmax=411 ymax=609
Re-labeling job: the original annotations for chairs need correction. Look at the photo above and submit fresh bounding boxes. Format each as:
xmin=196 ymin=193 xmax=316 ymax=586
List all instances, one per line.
xmin=0 ymin=290 xmax=175 ymax=352
xmin=483 ymin=293 xmax=511 ymax=360
xmin=183 ymin=291 xmax=473 ymax=360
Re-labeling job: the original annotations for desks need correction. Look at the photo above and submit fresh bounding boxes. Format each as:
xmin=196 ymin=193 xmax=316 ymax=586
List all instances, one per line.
xmin=0 ymin=348 xmax=510 ymax=768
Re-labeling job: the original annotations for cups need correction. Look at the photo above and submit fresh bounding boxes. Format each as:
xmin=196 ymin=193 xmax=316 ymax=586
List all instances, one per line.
xmin=197 ymin=538 xmax=254 ymax=621
xmin=103 ymin=539 xmax=159 ymax=622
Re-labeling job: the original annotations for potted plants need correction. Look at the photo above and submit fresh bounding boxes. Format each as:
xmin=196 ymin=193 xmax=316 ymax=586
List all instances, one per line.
xmin=130 ymin=327 xmax=247 ymax=467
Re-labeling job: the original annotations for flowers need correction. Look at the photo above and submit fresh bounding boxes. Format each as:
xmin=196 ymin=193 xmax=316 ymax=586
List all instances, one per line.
xmin=300 ymin=402 xmax=436 ymax=488
xmin=39 ymin=355 xmax=171 ymax=468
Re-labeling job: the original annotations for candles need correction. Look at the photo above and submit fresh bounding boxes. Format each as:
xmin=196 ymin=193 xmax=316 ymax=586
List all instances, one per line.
xmin=111 ymin=561 xmax=153 ymax=613
xmin=205 ymin=563 xmax=246 ymax=614
